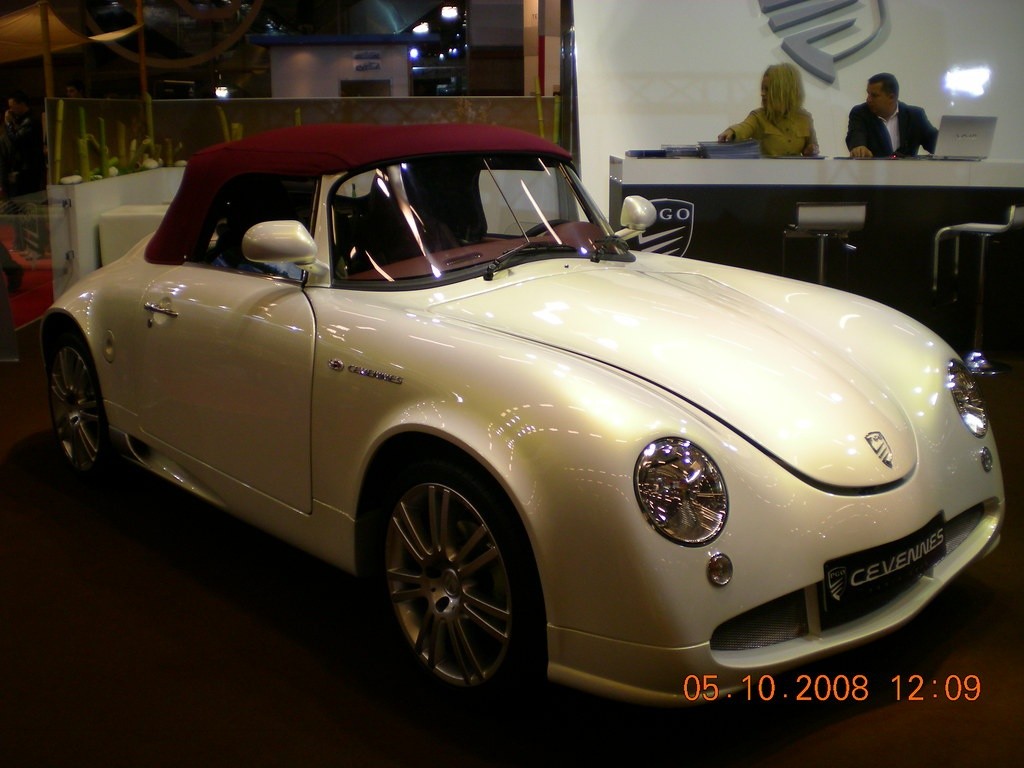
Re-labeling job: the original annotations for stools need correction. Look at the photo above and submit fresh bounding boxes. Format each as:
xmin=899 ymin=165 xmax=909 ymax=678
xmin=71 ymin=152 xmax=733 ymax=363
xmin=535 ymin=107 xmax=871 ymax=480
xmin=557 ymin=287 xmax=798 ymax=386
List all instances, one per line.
xmin=930 ymin=204 xmax=1024 ymax=351
xmin=780 ymin=199 xmax=868 ymax=285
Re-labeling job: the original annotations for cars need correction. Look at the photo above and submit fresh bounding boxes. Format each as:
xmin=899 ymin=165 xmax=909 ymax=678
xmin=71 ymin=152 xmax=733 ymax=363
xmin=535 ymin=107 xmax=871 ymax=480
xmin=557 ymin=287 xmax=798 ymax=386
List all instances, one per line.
xmin=39 ymin=122 xmax=1006 ymax=708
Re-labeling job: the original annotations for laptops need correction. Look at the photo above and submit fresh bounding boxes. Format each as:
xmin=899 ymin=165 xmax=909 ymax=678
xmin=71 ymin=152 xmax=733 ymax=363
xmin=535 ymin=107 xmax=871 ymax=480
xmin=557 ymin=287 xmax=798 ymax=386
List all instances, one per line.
xmin=912 ymin=115 xmax=998 ymax=164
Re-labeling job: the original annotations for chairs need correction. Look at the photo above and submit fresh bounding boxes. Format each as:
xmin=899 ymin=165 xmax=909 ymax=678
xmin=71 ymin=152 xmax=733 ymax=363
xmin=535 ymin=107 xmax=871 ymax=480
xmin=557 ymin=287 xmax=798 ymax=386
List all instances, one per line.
xmin=213 ymin=186 xmax=299 ymax=265
xmin=358 ymin=168 xmax=461 ymax=276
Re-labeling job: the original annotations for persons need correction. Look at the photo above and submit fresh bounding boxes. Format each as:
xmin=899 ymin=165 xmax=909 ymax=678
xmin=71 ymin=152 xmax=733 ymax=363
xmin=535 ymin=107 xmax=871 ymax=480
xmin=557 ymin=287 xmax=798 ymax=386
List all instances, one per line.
xmin=845 ymin=73 xmax=939 ymax=158
xmin=718 ymin=64 xmax=820 ymax=158
xmin=0 ymin=243 xmax=25 ymax=293
xmin=0 ymin=95 xmax=41 ymax=198
xmin=65 ymin=80 xmax=86 ymax=98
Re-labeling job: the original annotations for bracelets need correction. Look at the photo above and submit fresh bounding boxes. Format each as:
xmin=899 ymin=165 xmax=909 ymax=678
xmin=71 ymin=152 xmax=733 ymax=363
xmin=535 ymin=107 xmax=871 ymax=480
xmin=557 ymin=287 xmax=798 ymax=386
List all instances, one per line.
xmin=800 ymin=153 xmax=803 ymax=157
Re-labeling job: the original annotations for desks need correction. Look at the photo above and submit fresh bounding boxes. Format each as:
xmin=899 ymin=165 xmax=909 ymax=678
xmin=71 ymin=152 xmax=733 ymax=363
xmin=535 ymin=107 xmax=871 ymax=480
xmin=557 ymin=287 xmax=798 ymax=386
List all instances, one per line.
xmin=606 ymin=154 xmax=1024 ymax=357
